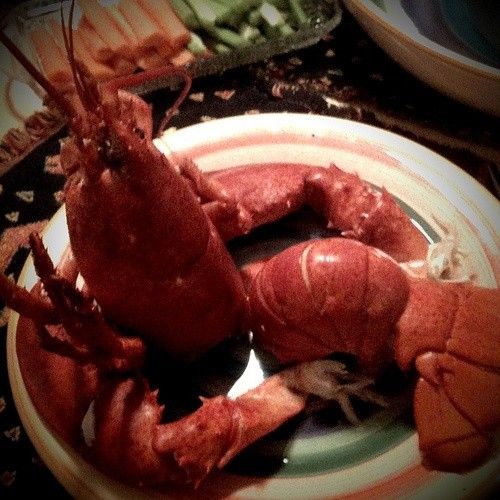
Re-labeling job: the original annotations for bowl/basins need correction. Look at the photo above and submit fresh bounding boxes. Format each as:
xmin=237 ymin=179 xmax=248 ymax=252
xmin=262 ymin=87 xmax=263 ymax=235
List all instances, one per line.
xmin=344 ymin=1 xmax=500 ymax=118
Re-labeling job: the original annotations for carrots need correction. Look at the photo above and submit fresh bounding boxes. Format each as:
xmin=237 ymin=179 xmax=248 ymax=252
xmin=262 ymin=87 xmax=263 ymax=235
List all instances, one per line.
xmin=31 ymin=0 xmax=197 ymax=96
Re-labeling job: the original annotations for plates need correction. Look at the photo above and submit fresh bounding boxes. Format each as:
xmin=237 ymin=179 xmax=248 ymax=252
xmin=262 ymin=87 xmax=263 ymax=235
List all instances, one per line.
xmin=9 ymin=0 xmax=343 ymax=115
xmin=8 ymin=112 xmax=500 ymax=499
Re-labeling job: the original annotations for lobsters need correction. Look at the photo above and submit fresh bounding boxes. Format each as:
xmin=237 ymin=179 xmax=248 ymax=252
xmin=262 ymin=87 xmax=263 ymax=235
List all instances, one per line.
xmin=1 ymin=0 xmax=499 ymax=488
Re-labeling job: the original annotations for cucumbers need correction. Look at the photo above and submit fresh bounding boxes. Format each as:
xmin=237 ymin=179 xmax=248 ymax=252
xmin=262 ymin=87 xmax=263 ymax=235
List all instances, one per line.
xmin=167 ymin=1 xmax=307 ymax=57
